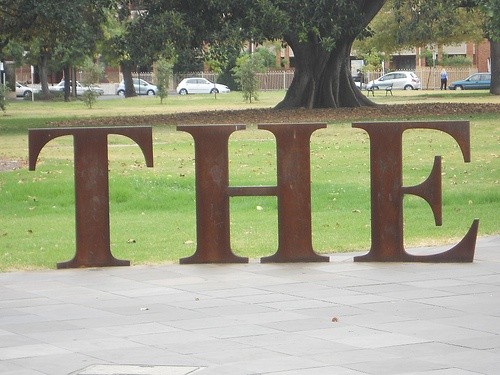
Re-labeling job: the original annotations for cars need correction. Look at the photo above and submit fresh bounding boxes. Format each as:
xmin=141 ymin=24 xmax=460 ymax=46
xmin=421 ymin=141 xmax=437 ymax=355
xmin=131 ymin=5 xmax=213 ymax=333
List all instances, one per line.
xmin=448 ymin=73 xmax=491 ymax=91
xmin=117 ymin=78 xmax=158 ymax=95
xmin=176 ymin=78 xmax=230 ymax=94
xmin=366 ymin=70 xmax=420 ymax=91
xmin=48 ymin=80 xmax=104 ymax=96
xmin=15 ymin=81 xmax=32 ymax=98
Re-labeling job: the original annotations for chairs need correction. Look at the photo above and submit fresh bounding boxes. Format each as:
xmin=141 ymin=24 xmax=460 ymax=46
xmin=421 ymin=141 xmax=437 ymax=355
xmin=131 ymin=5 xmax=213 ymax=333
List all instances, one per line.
xmin=366 ymin=85 xmax=375 ymax=97
xmin=384 ymin=83 xmax=394 ymax=96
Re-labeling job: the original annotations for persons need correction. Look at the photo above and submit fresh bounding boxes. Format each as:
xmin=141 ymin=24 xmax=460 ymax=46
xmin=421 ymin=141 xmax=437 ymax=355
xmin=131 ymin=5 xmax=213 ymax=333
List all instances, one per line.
xmin=440 ymin=68 xmax=448 ymax=91
xmin=356 ymin=68 xmax=362 ymax=77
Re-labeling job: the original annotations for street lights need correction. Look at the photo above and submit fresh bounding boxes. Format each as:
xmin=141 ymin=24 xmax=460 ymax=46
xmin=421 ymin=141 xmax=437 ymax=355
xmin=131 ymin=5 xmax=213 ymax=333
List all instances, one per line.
xmin=357 ymin=68 xmax=362 ymax=90
xmin=137 ymin=64 xmax=140 ymax=95
xmin=94 ymin=52 xmax=102 ymax=84
xmin=22 ymin=51 xmax=35 ymax=101
